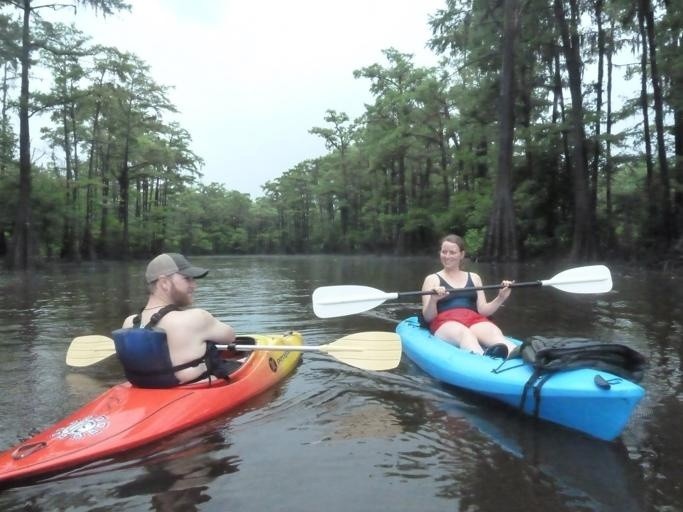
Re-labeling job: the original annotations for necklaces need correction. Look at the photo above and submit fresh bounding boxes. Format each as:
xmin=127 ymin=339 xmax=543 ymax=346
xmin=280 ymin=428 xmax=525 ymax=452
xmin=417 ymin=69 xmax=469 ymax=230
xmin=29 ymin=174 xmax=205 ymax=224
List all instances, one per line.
xmin=143 ymin=305 xmax=162 ymax=310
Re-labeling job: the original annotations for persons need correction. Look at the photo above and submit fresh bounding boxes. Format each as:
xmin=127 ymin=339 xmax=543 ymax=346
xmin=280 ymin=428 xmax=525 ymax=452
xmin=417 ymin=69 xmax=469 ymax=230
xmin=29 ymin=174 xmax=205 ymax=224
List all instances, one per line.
xmin=120 ymin=252 xmax=247 ymax=388
xmin=420 ymin=234 xmax=517 ymax=358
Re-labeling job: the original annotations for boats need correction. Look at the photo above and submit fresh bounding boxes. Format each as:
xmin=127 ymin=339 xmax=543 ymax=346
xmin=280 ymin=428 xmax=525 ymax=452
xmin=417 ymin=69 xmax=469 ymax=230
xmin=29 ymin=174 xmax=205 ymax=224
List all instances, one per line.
xmin=392 ymin=315 xmax=644 ymax=443
xmin=0 ymin=327 xmax=306 ymax=494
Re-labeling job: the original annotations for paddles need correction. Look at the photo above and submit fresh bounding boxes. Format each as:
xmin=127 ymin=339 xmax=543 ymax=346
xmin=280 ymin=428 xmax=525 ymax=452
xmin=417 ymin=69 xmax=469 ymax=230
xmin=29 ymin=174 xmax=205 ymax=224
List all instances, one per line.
xmin=66 ymin=331 xmax=402 ymax=371
xmin=312 ymin=264 xmax=614 ymax=319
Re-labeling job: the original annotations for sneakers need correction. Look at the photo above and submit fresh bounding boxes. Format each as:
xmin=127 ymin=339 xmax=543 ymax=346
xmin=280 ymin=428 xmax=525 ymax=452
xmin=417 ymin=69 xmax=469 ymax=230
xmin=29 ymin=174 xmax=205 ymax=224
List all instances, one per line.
xmin=482 ymin=342 xmax=509 ymax=358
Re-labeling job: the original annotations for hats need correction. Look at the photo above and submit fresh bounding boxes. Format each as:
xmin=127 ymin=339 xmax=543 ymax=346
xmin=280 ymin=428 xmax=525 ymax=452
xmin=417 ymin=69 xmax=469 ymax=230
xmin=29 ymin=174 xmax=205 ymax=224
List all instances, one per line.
xmin=143 ymin=252 xmax=209 ymax=284
